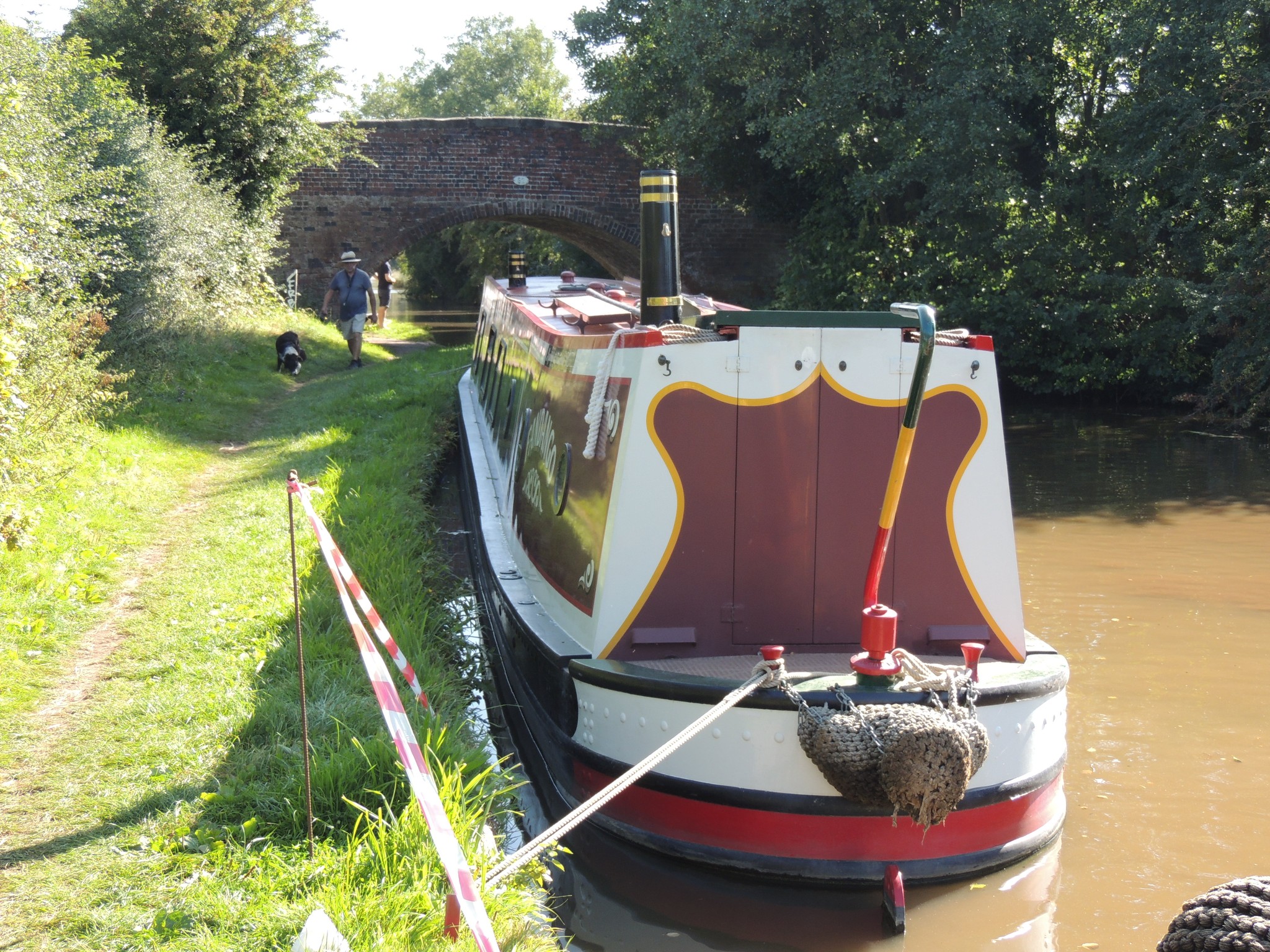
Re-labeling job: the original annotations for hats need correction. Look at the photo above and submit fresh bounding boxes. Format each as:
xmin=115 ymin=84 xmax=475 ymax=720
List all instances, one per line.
xmin=338 ymin=251 xmax=362 ymax=262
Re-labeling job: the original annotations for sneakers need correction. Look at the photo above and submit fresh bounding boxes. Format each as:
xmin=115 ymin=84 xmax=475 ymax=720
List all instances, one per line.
xmin=349 ymin=359 xmax=362 ymax=370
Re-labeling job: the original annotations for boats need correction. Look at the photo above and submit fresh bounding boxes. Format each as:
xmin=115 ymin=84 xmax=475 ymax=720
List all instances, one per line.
xmin=454 ymin=165 xmax=1068 ymax=932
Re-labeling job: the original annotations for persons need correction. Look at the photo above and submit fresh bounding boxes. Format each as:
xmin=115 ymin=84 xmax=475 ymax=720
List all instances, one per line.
xmin=321 ymin=250 xmax=378 ymax=367
xmin=372 ymin=261 xmax=396 ymax=330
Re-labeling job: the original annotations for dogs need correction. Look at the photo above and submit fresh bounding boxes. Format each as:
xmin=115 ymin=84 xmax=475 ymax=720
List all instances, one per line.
xmin=276 ymin=331 xmax=307 ymax=376
